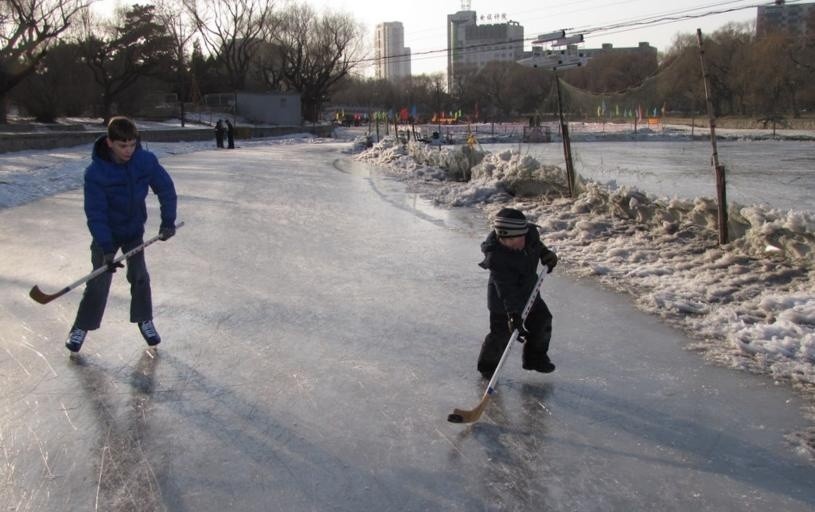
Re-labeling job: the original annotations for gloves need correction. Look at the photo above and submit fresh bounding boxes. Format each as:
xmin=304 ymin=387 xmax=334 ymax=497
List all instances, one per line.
xmin=103 ymin=252 xmax=124 ymax=273
xmin=507 ymin=313 xmax=527 ymax=343
xmin=540 ymin=250 xmax=557 ymax=273
xmin=159 ymin=225 xmax=175 ymax=240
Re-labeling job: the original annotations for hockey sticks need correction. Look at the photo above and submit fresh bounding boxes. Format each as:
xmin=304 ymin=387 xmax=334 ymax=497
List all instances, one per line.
xmin=448 ymin=265 xmax=549 ymax=423
xmin=29 ymin=221 xmax=182 ymax=304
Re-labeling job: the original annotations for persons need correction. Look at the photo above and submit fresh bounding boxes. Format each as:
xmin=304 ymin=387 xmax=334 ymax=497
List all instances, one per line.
xmin=224 ymin=119 xmax=235 ymax=149
xmin=473 ymin=206 xmax=559 ymax=383
xmin=213 ymin=119 xmax=226 ymax=149
xmin=62 ymin=115 xmax=178 ymax=353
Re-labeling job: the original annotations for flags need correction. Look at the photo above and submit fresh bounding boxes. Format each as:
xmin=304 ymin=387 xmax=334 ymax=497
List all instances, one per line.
xmin=598 ymin=97 xmax=668 ymax=125
xmin=333 ymin=101 xmax=484 ymax=124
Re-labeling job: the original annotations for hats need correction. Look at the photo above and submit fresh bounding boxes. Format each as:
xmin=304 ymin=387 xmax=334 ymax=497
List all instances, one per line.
xmin=495 ymin=208 xmax=529 ymax=238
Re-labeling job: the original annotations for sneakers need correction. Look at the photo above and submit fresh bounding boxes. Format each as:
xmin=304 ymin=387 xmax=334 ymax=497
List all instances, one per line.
xmin=64 ymin=325 xmax=87 ymax=351
xmin=522 ymin=360 xmax=555 ymax=372
xmin=138 ymin=319 xmax=161 ymax=346
xmin=481 ymin=370 xmax=495 ymax=379
